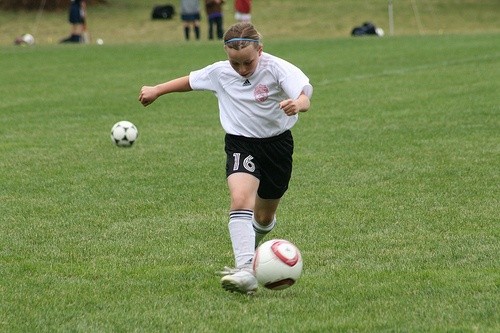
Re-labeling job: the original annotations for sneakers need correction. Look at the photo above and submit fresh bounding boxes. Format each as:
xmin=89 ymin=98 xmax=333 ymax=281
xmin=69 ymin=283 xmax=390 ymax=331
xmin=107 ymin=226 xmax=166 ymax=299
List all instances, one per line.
xmin=221 ymin=268 xmax=258 ymax=294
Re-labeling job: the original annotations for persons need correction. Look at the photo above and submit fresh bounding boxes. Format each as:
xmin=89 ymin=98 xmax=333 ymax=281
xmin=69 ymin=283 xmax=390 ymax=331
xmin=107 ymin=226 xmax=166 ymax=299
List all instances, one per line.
xmin=233 ymin=1 xmax=251 ymax=23
xmin=68 ymin=0 xmax=88 ymax=43
xmin=178 ymin=0 xmax=201 ymax=41
xmin=138 ymin=23 xmax=314 ymax=295
xmin=204 ymin=0 xmax=228 ymax=39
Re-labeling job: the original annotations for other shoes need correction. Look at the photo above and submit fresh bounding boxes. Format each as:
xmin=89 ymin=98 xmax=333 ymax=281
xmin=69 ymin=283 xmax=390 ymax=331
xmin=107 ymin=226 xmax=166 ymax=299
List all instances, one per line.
xmin=61 ymin=34 xmax=82 ymax=44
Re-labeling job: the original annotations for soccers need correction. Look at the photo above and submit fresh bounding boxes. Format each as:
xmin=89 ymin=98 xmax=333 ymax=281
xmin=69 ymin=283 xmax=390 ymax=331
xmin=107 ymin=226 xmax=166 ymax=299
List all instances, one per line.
xmin=252 ymin=239 xmax=304 ymax=291
xmin=110 ymin=120 xmax=139 ymax=149
